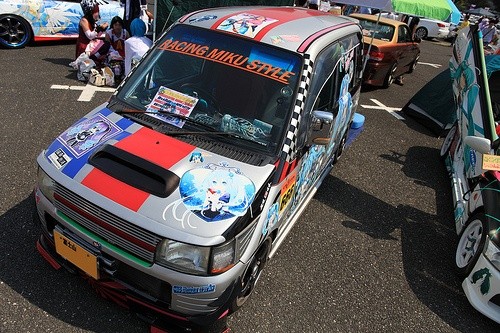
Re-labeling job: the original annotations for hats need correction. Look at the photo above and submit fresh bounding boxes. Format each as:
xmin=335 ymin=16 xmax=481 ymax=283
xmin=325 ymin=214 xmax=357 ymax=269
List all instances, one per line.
xmin=80 ymin=0 xmax=109 ymax=13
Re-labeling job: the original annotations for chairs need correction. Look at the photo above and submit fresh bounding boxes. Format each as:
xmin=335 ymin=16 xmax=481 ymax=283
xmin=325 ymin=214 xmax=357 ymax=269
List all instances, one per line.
xmin=259 ymin=66 xmax=325 ymax=143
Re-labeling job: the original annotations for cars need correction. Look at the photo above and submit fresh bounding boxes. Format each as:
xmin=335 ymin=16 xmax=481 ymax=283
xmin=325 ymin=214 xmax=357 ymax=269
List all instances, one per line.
xmin=374 ymin=12 xmax=451 ymax=41
xmin=350 ymin=13 xmax=421 ymax=88
xmin=0 ymin=1 xmax=150 ymax=49
xmin=33 ymin=7 xmax=365 ymax=327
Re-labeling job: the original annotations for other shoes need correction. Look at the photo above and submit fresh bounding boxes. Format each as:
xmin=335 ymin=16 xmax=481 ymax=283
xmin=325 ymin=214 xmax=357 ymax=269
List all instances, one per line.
xmin=395 ymin=76 xmax=404 ymax=86
xmin=449 ymin=44 xmax=453 ymax=46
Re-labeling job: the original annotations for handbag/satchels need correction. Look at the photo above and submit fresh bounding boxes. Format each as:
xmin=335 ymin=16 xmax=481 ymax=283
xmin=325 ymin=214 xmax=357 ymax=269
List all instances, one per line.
xmin=81 ymin=66 xmax=114 ymax=86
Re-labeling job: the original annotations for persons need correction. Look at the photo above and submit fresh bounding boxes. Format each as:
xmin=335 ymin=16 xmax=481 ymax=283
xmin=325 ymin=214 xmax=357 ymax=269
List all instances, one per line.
xmin=75 ymin=3 xmax=154 ymax=87
xmin=477 ymin=13 xmax=500 ymax=55
xmin=448 ymin=14 xmax=471 ymax=47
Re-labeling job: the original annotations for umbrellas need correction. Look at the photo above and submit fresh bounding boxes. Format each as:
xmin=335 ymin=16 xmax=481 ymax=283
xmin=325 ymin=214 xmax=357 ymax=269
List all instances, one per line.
xmin=330 ymin=0 xmax=462 ymax=74
